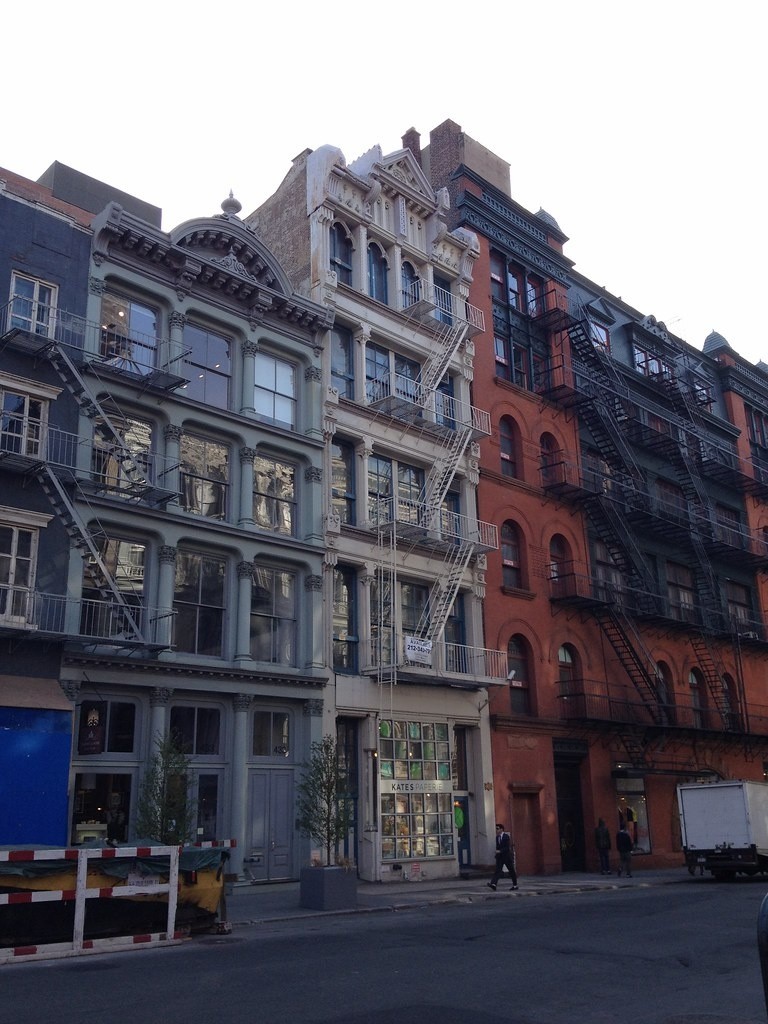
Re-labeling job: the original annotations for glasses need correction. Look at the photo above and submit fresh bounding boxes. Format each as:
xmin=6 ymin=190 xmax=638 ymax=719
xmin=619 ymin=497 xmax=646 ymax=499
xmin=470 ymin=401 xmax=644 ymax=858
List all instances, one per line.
xmin=496 ymin=828 xmax=500 ymax=830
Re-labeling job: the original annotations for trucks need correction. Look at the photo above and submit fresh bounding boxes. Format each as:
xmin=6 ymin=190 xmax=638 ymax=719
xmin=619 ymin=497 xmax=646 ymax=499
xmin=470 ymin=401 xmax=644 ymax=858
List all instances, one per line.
xmin=677 ymin=780 xmax=768 ymax=882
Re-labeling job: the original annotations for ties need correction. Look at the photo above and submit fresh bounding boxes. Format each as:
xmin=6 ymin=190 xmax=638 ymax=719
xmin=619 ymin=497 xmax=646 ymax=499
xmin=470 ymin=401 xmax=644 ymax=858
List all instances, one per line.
xmin=500 ymin=836 xmax=501 ymax=844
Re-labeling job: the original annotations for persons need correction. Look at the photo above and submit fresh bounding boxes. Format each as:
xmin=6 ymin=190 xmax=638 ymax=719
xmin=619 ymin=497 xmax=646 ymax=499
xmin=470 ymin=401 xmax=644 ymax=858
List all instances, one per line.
xmin=594 ymin=818 xmax=612 ymax=876
xmin=617 ymin=823 xmax=633 ymax=878
xmin=486 ymin=824 xmax=519 ymax=890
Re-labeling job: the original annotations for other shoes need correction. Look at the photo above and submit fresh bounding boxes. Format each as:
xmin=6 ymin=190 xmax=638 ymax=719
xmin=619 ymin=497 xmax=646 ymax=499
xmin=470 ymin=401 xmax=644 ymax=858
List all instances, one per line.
xmin=626 ymin=874 xmax=632 ymax=878
xmin=602 ymin=871 xmax=606 ymax=875
xmin=487 ymin=883 xmax=496 ymax=890
xmin=608 ymin=871 xmax=612 ymax=874
xmin=617 ymin=868 xmax=621 ymax=876
xmin=509 ymin=887 xmax=518 ymax=890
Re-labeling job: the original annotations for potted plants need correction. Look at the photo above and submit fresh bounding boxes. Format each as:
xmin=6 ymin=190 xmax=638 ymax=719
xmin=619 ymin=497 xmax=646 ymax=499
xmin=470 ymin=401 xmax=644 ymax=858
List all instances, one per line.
xmin=289 ymin=735 xmax=359 ymax=911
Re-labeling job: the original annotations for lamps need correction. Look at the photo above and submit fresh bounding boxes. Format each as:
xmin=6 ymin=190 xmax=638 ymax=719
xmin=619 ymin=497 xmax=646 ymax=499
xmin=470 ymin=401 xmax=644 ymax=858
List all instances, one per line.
xmin=89 ymin=708 xmax=99 ymax=725
